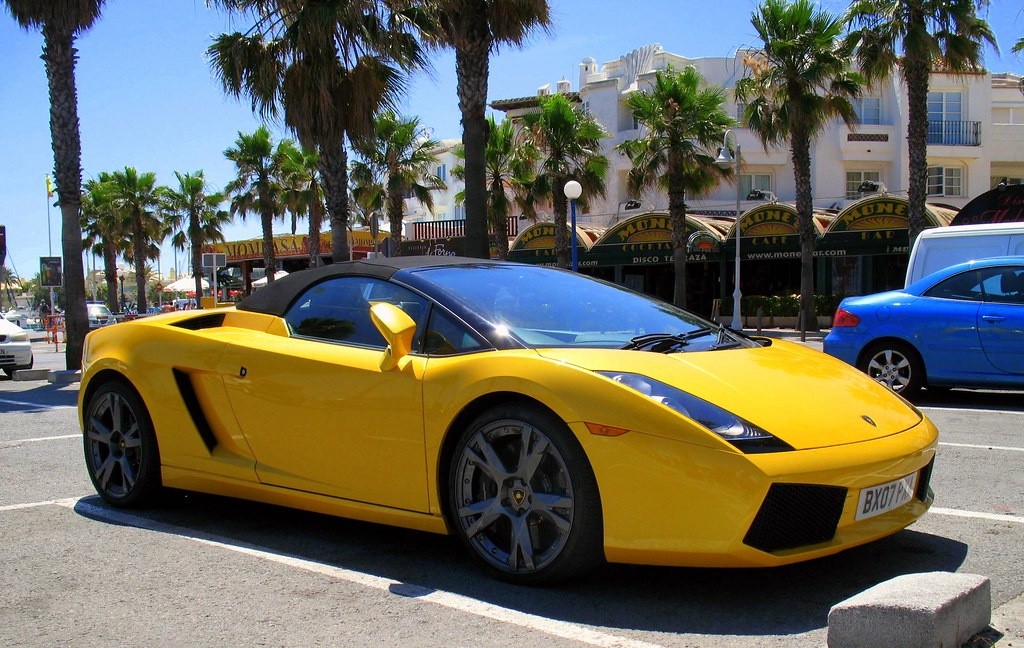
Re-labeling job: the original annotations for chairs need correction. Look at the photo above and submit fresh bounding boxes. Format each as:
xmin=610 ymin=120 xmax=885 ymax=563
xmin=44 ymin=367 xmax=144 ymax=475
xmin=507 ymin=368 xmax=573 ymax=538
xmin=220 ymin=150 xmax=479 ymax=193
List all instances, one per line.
xmin=308 ymin=283 xmax=372 ymax=333
xmin=1000 ymin=271 xmax=1024 ymax=293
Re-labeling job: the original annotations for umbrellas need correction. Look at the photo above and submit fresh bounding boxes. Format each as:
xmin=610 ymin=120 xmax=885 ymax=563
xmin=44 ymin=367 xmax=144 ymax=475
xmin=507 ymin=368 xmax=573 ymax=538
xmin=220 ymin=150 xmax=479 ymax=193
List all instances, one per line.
xmin=163 ymin=276 xmax=209 ymax=293
xmin=251 ymin=270 xmax=289 ymax=288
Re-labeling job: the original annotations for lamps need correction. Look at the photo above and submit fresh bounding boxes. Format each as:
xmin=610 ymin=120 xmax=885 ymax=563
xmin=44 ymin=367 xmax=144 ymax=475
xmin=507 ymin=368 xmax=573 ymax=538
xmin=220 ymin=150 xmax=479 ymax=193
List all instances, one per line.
xmin=519 ymin=210 xmax=552 ymax=223
xmin=625 ymin=199 xmax=657 ymax=211
xmin=858 ymin=180 xmax=892 ymax=196
xmin=746 ymin=189 xmax=779 ymax=203
xmin=998 ymin=181 xmax=1020 ymax=190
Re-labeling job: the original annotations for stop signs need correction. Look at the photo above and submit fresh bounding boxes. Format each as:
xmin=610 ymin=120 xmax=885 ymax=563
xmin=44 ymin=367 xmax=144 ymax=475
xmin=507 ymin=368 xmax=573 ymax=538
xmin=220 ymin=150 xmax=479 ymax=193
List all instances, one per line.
xmin=155 ymin=282 xmax=162 ymax=291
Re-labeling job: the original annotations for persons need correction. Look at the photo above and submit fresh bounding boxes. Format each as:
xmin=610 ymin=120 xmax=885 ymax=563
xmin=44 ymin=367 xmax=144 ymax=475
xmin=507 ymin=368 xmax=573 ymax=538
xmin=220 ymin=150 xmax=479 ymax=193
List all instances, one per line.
xmin=154 ymin=301 xmax=196 ymax=314
xmin=39 ymin=299 xmax=51 ymax=329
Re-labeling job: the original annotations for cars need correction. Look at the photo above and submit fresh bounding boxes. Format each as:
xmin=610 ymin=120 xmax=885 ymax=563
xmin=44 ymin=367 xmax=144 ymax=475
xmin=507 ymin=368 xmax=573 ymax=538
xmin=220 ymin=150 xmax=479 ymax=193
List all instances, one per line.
xmin=86 ymin=304 xmax=117 ymax=327
xmin=0 ymin=310 xmax=34 ymax=375
xmin=823 ymin=253 xmax=1023 ymax=401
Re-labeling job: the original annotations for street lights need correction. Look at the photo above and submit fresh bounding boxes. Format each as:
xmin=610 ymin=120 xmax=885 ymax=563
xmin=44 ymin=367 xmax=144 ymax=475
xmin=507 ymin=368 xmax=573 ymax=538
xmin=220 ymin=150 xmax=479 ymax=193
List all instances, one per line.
xmin=563 ymin=180 xmax=583 ymax=272
xmin=711 ymin=129 xmax=746 ymax=334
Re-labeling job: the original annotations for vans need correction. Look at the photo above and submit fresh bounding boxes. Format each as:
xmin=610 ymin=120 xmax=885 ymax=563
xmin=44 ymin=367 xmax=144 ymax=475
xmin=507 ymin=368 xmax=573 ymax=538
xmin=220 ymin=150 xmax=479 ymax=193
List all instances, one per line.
xmin=900 ymin=221 xmax=1024 ymax=291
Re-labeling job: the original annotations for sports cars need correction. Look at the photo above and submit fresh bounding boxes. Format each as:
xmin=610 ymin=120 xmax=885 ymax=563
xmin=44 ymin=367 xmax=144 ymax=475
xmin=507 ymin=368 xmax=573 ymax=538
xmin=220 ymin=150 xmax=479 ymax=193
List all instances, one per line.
xmin=77 ymin=255 xmax=940 ymax=589
